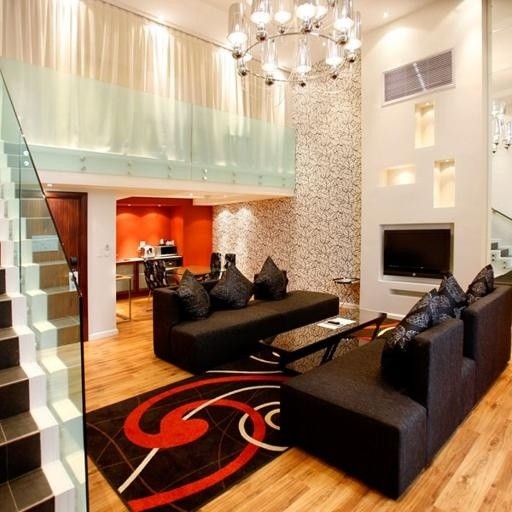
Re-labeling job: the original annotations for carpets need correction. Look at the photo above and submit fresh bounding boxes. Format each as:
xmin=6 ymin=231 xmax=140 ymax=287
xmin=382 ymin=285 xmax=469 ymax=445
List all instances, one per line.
xmin=64 ymin=319 xmax=402 ymax=512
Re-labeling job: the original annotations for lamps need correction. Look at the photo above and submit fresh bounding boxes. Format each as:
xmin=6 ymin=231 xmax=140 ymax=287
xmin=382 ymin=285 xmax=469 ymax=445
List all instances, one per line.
xmin=226 ymin=1 xmax=363 ymax=88
xmin=492 ymin=101 xmax=511 ymax=156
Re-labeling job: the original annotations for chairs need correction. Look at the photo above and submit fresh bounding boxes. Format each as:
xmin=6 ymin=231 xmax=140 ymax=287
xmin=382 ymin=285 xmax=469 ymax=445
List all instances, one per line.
xmin=144 ymin=252 xmax=236 ymax=312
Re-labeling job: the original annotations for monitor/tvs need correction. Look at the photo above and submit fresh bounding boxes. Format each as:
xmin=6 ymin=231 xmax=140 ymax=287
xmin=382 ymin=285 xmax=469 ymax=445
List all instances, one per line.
xmin=382 ymin=228 xmax=451 ymax=280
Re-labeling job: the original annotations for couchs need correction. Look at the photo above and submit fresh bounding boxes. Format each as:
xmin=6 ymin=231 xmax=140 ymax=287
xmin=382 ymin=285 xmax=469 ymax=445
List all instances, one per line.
xmin=280 ymin=271 xmax=512 ymax=500
xmin=152 ymin=277 xmax=339 ymax=376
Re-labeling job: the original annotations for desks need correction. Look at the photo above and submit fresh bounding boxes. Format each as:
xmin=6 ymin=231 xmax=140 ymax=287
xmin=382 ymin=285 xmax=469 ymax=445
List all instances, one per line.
xmin=167 ymin=266 xmax=228 ymax=279
xmin=114 ymin=257 xmax=148 ymax=295
xmin=116 ymin=276 xmax=133 ymax=326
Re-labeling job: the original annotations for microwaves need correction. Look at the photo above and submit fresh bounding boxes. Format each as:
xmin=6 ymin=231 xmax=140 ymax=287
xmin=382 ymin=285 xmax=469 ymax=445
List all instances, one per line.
xmin=154 ymin=245 xmax=178 ymax=257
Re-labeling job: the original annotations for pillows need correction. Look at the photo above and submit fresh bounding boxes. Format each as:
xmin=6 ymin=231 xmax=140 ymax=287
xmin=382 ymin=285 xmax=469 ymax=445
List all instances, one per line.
xmin=379 ymin=289 xmax=437 ymax=350
xmin=468 ymin=264 xmax=496 ymax=304
xmin=178 ymin=268 xmax=210 ymax=317
xmin=440 ymin=272 xmax=468 ymax=318
xmin=255 ymin=254 xmax=289 ymax=300
xmin=212 ymin=263 xmax=254 ymax=307
